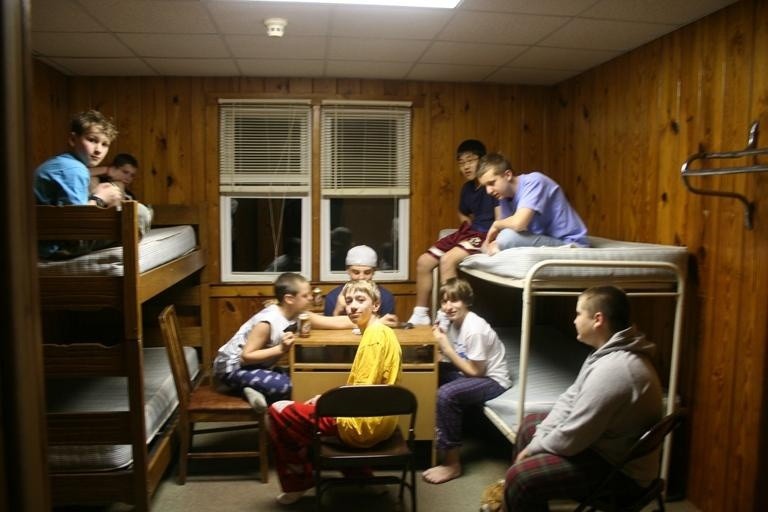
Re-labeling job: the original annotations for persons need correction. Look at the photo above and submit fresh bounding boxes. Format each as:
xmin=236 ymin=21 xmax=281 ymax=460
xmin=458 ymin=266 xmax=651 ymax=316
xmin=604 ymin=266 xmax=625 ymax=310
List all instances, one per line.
xmin=89 ymin=154 xmax=138 ymax=201
xmin=210 ymin=273 xmax=398 ymax=409
xmin=504 ymin=287 xmax=663 ymax=511
xmin=324 ymin=246 xmax=396 ymax=318
xmin=476 ymin=152 xmax=590 ymax=257
xmin=422 ymin=279 xmax=513 ymax=483
xmin=406 ymin=140 xmax=501 ymax=326
xmin=264 ymin=279 xmax=403 ymax=505
xmin=34 ymin=111 xmax=144 ymax=261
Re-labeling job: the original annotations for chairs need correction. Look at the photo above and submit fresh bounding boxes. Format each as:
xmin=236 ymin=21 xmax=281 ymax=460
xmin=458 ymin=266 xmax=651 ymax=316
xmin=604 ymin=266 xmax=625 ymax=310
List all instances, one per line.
xmin=157 ymin=303 xmax=272 ymax=486
xmin=310 ymin=383 xmax=420 ymax=512
xmin=579 ymin=415 xmax=683 ymax=512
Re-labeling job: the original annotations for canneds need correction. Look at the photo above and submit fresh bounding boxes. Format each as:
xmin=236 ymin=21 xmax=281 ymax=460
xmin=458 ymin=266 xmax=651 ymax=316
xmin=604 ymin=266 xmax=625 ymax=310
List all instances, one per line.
xmin=312 ymin=288 xmax=322 ymax=306
xmin=297 ymin=313 xmax=311 ymax=337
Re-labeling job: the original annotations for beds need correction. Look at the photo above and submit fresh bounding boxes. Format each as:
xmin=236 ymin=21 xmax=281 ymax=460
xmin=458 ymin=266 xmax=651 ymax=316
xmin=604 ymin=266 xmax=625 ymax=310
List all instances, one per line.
xmin=430 ymin=228 xmax=690 ymax=512
xmin=32 ymin=200 xmax=216 ymax=512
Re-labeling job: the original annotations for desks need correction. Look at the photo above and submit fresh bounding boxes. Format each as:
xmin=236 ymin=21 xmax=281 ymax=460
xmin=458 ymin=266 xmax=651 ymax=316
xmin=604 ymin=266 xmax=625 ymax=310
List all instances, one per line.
xmin=288 ymin=324 xmax=440 ymax=472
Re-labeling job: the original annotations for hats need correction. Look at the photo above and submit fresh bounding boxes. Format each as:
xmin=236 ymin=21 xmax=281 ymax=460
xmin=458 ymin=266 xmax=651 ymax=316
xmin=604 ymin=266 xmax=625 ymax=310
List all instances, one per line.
xmin=345 ymin=244 xmax=378 ymax=267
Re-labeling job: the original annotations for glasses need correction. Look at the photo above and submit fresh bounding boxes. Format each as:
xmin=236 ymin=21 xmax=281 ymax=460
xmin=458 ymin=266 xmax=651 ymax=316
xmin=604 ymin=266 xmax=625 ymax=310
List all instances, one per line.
xmin=457 ymin=157 xmax=480 ymax=165
